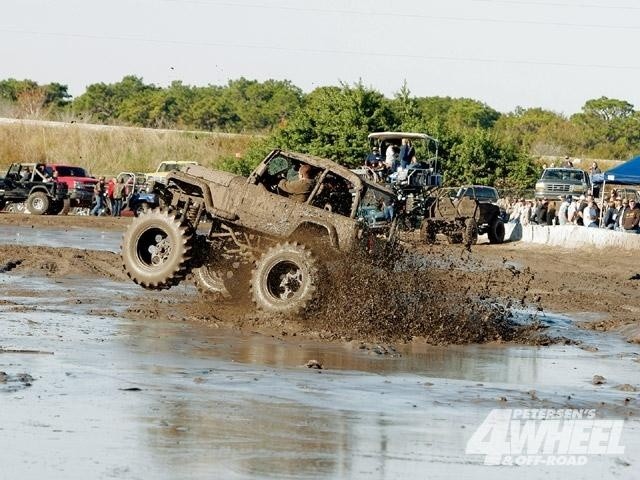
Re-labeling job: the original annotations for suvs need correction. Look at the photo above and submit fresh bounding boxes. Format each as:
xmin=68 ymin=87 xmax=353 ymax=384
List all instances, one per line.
xmin=420 ymin=185 xmax=505 ymax=245
xmin=1 ymin=162 xmax=68 ymax=216
xmin=536 ymin=167 xmax=590 ymax=200
xmin=49 ymin=164 xmax=99 ymax=201
xmin=130 ymin=161 xmax=197 ymax=216
xmin=119 ymin=150 xmax=398 ymax=320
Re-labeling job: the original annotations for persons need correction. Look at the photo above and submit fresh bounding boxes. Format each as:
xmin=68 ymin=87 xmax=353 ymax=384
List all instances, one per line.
xmin=279 ymin=165 xmax=316 ymax=203
xmin=589 ymin=162 xmax=603 ymax=198
xmin=499 ymin=190 xmax=640 ymax=237
xmin=379 ymin=184 xmax=396 ymax=222
xmin=313 ymin=176 xmax=352 ymax=217
xmin=364 ymin=138 xmax=421 ymax=186
xmin=561 ymin=156 xmax=574 ymax=169
xmin=15 ymin=165 xmax=138 ymax=217
xmin=588 ymin=167 xmax=594 ymax=196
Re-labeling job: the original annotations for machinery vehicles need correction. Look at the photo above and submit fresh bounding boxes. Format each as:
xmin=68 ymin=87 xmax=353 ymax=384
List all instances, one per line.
xmin=346 ymin=131 xmax=444 ymax=232
